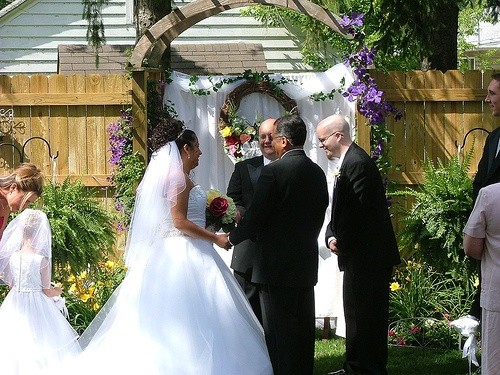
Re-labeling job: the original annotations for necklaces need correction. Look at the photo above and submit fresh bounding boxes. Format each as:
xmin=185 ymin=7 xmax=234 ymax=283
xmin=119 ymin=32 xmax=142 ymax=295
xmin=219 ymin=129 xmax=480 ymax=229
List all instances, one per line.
xmin=0 ymin=199 xmax=9 ymax=210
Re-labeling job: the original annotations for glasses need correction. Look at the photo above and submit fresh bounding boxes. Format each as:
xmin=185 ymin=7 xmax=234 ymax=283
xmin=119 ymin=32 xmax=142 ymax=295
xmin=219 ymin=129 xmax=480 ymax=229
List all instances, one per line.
xmin=320 ymin=130 xmax=344 ymax=145
xmin=268 ymin=133 xmax=293 ymax=146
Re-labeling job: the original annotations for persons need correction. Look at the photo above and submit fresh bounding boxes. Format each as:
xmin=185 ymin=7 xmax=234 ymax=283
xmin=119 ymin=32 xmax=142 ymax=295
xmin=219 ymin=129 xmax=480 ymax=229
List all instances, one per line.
xmin=0 ymin=162 xmax=82 ymax=375
xmin=462 ymin=72 xmax=500 ymax=375
xmin=316 ymin=115 xmax=401 ymax=375
xmin=226 ymin=115 xmax=329 ymax=375
xmin=60 ymin=116 xmax=273 ymax=375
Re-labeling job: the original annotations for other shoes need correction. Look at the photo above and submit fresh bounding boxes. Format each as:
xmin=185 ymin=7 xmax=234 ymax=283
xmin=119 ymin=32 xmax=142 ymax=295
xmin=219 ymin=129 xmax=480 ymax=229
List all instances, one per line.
xmin=328 ymin=370 xmax=347 ymax=375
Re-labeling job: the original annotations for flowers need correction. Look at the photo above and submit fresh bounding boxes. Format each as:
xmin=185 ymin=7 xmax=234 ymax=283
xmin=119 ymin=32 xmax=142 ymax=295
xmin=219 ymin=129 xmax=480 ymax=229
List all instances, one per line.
xmin=388 ymin=327 xmax=421 ymax=346
xmin=339 ymin=12 xmax=403 ymax=207
xmin=68 ymin=261 xmax=116 ymax=310
xmin=107 ymin=109 xmax=136 ymax=166
xmin=216 ymin=126 xmax=253 ymax=161
xmin=106 ymin=192 xmax=128 ymax=233
xmin=205 ymin=191 xmax=230 ymax=217
xmin=389 ymin=281 xmax=401 ymax=293
